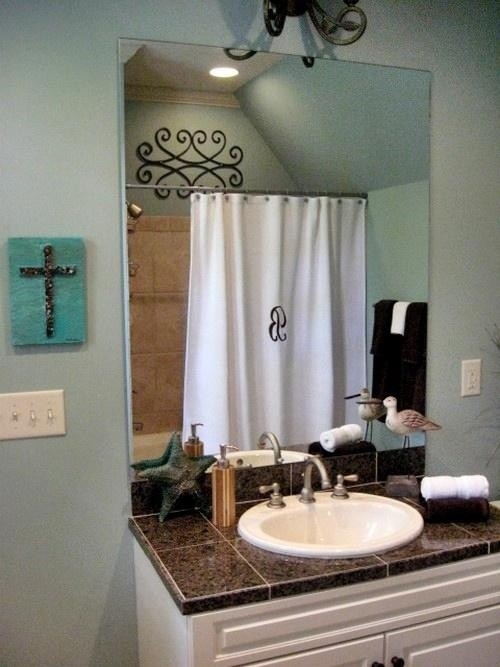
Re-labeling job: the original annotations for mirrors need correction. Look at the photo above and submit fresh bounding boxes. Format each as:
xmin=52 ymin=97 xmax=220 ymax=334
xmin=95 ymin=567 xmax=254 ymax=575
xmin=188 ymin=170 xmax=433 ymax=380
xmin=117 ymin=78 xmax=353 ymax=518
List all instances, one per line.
xmin=114 ymin=35 xmax=435 ymax=484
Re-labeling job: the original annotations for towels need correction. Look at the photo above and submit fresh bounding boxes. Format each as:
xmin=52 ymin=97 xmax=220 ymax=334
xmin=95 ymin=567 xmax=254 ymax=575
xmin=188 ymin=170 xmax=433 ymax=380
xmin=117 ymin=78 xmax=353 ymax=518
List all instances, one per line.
xmin=419 ymin=473 xmax=493 ymax=522
xmin=307 ymin=419 xmax=377 ymax=461
xmin=390 ymin=300 xmax=412 ymax=336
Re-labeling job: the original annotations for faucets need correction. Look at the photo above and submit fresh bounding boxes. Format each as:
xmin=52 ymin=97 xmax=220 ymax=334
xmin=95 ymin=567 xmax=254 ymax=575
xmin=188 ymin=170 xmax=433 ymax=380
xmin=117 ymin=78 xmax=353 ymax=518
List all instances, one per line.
xmin=297 ymin=452 xmax=333 ymax=503
xmin=258 ymin=430 xmax=287 ymax=465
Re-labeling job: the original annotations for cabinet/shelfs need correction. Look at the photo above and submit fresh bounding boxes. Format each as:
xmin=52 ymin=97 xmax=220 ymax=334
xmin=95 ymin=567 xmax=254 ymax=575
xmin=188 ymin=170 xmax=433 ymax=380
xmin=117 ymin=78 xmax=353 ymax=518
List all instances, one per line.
xmin=130 ymin=531 xmax=500 ymax=667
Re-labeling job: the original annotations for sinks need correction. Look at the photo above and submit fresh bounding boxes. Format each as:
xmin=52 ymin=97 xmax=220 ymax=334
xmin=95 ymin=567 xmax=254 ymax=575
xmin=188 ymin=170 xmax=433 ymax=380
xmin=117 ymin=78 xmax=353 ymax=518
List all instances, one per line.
xmin=233 ymin=486 xmax=426 ymax=560
xmin=205 ymin=448 xmax=313 ymax=471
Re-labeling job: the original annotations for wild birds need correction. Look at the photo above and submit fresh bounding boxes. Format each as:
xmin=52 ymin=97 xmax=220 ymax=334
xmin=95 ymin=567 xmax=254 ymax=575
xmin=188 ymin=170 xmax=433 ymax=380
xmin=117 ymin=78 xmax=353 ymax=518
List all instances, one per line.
xmin=356 ymin=396 xmax=443 ymax=451
xmin=344 ymin=388 xmax=387 ymax=445
